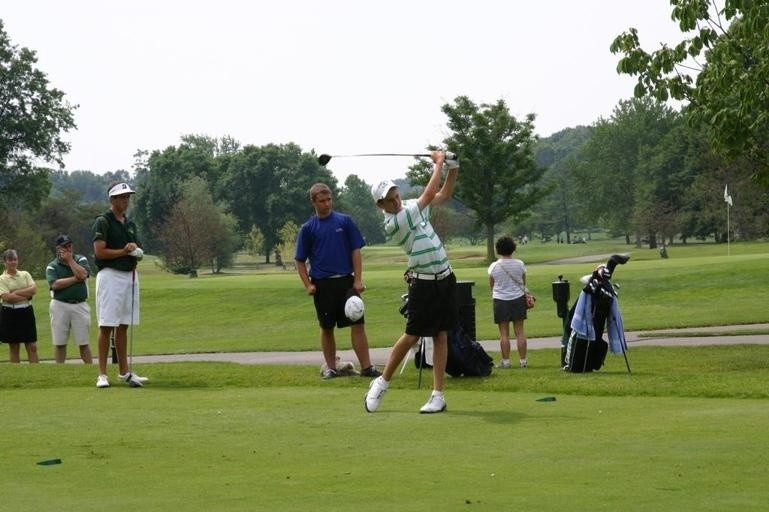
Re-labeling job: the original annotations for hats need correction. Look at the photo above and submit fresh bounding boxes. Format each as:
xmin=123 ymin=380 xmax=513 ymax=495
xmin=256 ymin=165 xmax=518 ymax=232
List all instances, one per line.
xmin=108 ymin=182 xmax=136 ymax=198
xmin=56 ymin=235 xmax=72 ymax=249
xmin=371 ymin=179 xmax=400 ymax=203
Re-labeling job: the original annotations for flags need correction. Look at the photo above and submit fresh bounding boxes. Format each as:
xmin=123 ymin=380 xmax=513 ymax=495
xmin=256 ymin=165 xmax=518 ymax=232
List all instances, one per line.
xmin=723 ymin=183 xmax=734 ymax=207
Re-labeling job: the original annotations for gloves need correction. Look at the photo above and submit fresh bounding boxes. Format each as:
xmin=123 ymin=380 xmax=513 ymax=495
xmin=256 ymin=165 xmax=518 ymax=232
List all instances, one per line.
xmin=444 ymin=151 xmax=460 ymax=170
xmin=127 ymin=247 xmax=143 ymax=258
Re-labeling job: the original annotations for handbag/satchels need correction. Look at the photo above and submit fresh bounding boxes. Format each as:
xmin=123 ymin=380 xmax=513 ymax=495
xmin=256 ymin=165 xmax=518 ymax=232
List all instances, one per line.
xmin=525 ymin=293 xmax=536 ymax=308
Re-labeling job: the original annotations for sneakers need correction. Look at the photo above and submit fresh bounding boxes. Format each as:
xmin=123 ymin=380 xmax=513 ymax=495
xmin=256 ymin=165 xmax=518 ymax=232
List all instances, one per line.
xmin=96 ymin=375 xmax=111 ymax=388
xmin=322 ymin=369 xmax=339 ymax=379
xmin=494 ymin=359 xmax=512 ymax=369
xmin=519 ymin=358 xmax=528 ymax=367
xmin=117 ymin=371 xmax=148 ymax=383
xmin=364 ymin=375 xmax=391 ymax=413
xmin=360 ymin=365 xmax=382 ymax=377
xmin=419 ymin=392 xmax=447 ymax=413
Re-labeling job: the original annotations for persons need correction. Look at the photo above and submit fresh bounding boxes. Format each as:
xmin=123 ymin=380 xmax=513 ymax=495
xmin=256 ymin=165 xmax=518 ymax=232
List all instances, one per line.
xmin=486 ymin=235 xmax=530 ymax=370
xmin=291 ymin=181 xmax=383 ymax=381
xmin=91 ymin=180 xmax=152 ymax=389
xmin=360 ymin=149 xmax=463 ymax=414
xmin=0 ymin=246 xmax=41 ymax=367
xmin=516 ymin=232 xmax=589 ymax=245
xmin=44 ymin=231 xmax=96 ymax=367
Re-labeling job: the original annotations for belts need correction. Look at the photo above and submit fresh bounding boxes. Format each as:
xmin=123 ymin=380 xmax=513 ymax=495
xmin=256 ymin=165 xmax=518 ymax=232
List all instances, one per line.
xmin=52 ymin=297 xmax=85 ymax=304
xmin=327 ymin=274 xmax=348 ymax=279
xmin=407 ymin=266 xmax=453 ymax=281
xmin=2 ymin=300 xmax=32 ymax=309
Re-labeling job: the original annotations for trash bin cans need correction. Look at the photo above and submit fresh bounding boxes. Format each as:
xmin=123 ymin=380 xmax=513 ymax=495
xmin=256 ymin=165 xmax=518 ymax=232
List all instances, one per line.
xmin=456 ymin=281 xmax=476 ymax=342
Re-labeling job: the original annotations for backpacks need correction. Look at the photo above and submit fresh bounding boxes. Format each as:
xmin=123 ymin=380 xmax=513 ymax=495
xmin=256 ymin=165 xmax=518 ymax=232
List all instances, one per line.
xmin=444 ymin=326 xmax=495 ymax=378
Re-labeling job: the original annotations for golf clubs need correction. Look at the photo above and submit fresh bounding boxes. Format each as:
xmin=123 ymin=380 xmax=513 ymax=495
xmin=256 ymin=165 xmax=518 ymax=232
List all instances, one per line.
xmin=125 ymin=259 xmax=142 ymax=386
xmin=318 ymin=152 xmax=456 ymax=165
